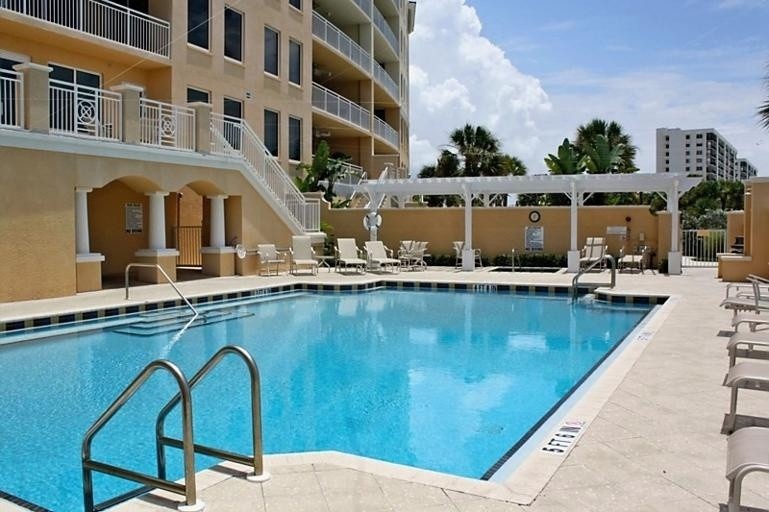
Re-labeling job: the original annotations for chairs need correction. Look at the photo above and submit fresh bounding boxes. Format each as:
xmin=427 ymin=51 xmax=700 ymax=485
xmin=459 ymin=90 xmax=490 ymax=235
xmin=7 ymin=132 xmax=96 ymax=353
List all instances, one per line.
xmin=617 ymin=246 xmax=649 ymax=275
xmin=575 ymin=236 xmax=608 ymax=272
xmin=718 ymin=273 xmax=769 ymax=512
xmin=254 ymin=235 xmax=483 ymax=278
